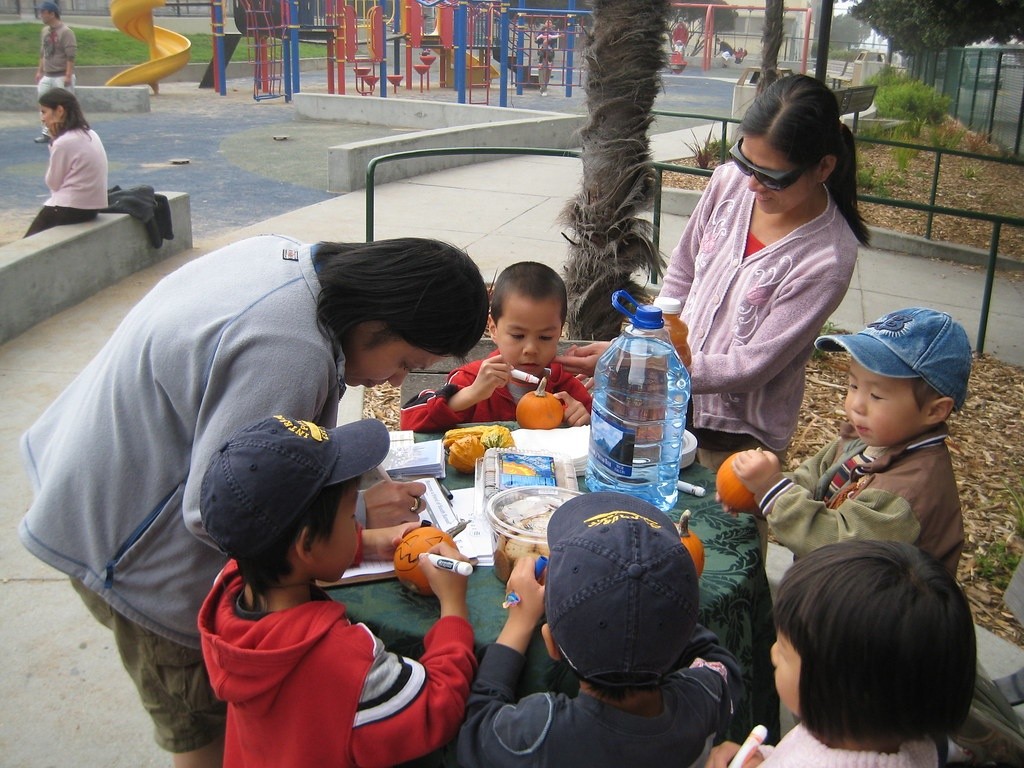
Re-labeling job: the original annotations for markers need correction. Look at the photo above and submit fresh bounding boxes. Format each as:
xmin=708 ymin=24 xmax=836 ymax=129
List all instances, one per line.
xmin=677 ymin=480 xmax=706 ymax=498
xmin=511 ymin=369 xmax=542 ymax=385
xmin=728 ymin=725 xmax=768 ymax=768
xmin=534 ymin=555 xmax=549 ymax=580
xmin=440 ymin=481 xmax=454 ymax=501
xmin=419 ymin=551 xmax=474 ymax=576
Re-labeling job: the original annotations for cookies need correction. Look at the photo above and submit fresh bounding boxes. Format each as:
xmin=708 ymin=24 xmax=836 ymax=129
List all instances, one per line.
xmin=492 ymin=507 xmax=552 ymax=586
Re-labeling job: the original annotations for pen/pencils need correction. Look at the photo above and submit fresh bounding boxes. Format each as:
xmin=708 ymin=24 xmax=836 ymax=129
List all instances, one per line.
xmin=376 ymin=464 xmax=394 ymax=483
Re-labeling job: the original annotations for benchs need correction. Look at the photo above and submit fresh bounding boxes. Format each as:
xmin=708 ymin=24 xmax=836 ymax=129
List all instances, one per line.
xmin=834 ymin=86 xmax=877 ymax=135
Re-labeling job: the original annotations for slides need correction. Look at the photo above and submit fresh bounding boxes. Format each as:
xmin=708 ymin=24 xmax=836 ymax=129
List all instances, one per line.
xmin=102 ymin=2 xmax=192 ymax=92
xmin=431 ymin=41 xmax=500 ymax=81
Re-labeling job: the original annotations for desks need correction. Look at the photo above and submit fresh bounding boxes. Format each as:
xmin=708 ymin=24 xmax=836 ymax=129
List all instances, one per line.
xmin=310 ymin=420 xmax=781 ymax=750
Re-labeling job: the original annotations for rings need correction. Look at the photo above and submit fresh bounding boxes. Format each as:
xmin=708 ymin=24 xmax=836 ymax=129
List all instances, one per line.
xmin=410 ymin=497 xmax=421 ymax=512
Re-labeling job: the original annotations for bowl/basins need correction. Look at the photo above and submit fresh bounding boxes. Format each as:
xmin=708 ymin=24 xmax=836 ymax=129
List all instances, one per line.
xmin=484 ymin=485 xmax=587 ymax=586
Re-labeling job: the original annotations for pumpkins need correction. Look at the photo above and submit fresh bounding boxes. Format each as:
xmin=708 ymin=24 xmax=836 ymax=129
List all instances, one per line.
xmin=716 ymin=447 xmax=762 ymax=512
xmin=441 ymin=425 xmax=516 ymax=473
xmin=672 ymin=509 xmax=705 ymax=581
xmin=394 ymin=522 xmax=467 ymax=595
xmin=516 ymin=376 xmax=564 ymax=429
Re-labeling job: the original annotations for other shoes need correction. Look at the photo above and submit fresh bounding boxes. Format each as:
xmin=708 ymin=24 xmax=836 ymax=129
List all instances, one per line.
xmin=35 ymin=134 xmax=49 ymax=143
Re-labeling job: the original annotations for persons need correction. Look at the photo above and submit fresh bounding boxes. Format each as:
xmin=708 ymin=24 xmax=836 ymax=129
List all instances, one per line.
xmin=32 ymin=1 xmax=77 ymax=142
xmin=23 ymin=87 xmax=109 ymax=236
xmin=714 ymin=38 xmax=734 ymax=69
xmin=716 ymin=309 xmax=974 ymax=583
xmin=732 ymin=46 xmax=747 ymax=64
xmin=399 ymin=262 xmax=593 ymax=433
xmin=702 ymin=538 xmax=976 ymax=768
xmin=18 ymin=233 xmax=488 ymax=768
xmin=674 ymin=40 xmax=685 ymax=55
xmin=552 ymin=75 xmax=871 ymax=473
xmin=197 ymin=414 xmax=745 ymax=767
xmin=536 ymin=27 xmax=562 ymax=71
xmin=672 ymin=17 xmax=690 ymax=59
xmin=534 ymin=18 xmax=565 ymax=97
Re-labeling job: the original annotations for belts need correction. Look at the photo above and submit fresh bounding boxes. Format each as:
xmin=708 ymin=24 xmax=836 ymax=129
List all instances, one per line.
xmin=45 ymin=71 xmax=65 ymax=77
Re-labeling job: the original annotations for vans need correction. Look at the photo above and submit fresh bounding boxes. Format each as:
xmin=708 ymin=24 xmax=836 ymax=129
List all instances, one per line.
xmin=959 ymin=54 xmax=1004 ymax=91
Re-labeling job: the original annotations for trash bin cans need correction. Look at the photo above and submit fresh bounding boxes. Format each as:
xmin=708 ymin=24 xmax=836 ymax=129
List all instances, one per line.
xmin=730 ymin=68 xmax=795 ymax=143
xmin=805 ymin=68 xmax=831 ymax=89
xmin=852 ymin=50 xmax=886 ymax=87
xmin=892 ymin=51 xmax=911 ymax=74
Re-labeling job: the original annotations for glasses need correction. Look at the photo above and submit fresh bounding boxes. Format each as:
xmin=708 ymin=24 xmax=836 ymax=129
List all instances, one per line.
xmin=729 ymin=135 xmax=807 ymax=191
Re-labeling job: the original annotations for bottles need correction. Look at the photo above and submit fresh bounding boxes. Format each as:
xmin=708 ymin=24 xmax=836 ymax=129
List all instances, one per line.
xmin=584 ymin=289 xmax=691 ymax=514
xmin=652 ymin=297 xmax=692 ymax=379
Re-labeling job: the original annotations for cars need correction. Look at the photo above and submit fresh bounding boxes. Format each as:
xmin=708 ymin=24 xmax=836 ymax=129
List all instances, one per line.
xmin=935 ymin=51 xmax=949 ymax=79
xmin=998 ymin=52 xmax=1021 ymax=68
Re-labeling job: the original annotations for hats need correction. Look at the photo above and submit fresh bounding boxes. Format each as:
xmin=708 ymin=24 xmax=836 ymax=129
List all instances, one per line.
xmin=31 ymin=0 xmax=61 ymax=15
xmin=199 ymin=415 xmax=389 ymax=555
xmin=546 ymin=491 xmax=699 ymax=688
xmin=814 ymin=309 xmax=973 ymax=413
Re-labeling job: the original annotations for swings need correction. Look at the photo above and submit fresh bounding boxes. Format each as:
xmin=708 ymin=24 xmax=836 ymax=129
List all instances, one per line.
xmin=733 ymin=9 xmax=752 ymax=63
